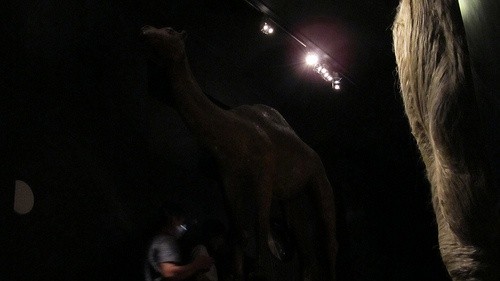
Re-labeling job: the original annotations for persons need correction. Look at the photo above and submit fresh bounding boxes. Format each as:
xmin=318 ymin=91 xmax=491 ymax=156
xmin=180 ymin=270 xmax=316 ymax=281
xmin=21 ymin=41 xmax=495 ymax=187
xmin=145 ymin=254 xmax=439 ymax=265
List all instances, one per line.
xmin=142 ymin=212 xmax=220 ymax=281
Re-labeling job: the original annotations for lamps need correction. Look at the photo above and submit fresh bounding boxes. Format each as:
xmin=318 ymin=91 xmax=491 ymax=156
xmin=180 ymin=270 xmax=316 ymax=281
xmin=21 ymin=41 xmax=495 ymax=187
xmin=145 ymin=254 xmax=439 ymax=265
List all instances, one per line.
xmin=301 ymin=46 xmax=344 ymax=92
xmin=257 ymin=17 xmax=275 ymax=37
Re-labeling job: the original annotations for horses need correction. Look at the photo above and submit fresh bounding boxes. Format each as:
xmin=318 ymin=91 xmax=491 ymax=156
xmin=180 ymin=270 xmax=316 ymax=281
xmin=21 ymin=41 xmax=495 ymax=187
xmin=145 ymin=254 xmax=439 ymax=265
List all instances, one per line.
xmin=390 ymin=0 xmax=500 ymax=281
xmin=136 ymin=24 xmax=339 ymax=281
